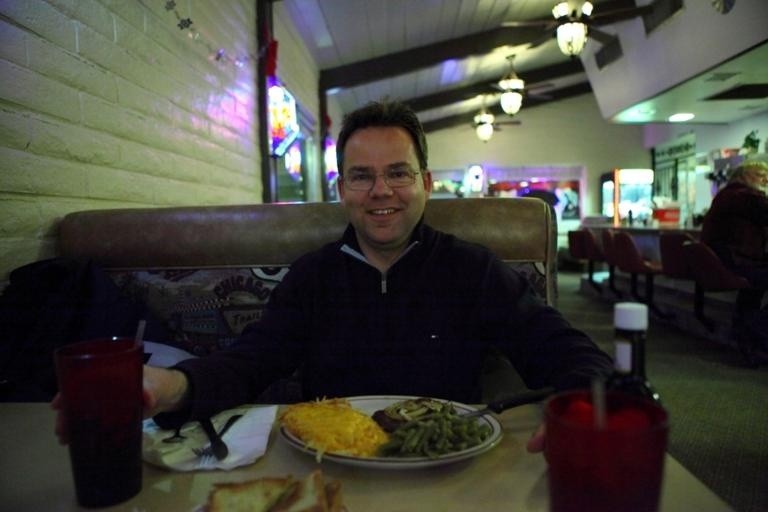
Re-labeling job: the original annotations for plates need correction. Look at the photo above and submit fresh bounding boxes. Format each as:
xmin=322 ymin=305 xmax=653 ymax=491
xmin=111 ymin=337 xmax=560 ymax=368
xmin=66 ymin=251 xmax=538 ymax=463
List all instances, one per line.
xmin=278 ymin=394 xmax=504 ymax=470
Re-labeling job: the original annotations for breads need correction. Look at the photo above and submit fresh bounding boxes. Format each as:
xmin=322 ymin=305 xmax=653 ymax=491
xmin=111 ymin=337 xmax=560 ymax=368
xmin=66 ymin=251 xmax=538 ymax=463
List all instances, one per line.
xmin=208 ymin=469 xmax=344 ymax=510
xmin=278 ymin=396 xmax=387 ymax=464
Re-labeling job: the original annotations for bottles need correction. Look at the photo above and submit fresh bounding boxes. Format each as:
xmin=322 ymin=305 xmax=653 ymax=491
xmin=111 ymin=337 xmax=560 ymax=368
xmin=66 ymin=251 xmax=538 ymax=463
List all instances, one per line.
xmin=601 ymin=302 xmax=663 ymax=406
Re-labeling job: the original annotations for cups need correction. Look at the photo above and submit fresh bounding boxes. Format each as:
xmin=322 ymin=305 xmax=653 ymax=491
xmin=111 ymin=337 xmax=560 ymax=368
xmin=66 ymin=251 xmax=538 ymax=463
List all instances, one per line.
xmin=52 ymin=339 xmax=146 ymax=508
xmin=545 ymin=386 xmax=669 ymax=511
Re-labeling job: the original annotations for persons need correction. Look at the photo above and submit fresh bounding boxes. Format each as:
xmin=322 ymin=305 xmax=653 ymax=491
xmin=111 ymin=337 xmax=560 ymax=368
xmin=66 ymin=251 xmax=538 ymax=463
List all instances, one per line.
xmin=49 ymin=93 xmax=614 ymax=463
xmin=699 ymin=156 xmax=766 ymax=373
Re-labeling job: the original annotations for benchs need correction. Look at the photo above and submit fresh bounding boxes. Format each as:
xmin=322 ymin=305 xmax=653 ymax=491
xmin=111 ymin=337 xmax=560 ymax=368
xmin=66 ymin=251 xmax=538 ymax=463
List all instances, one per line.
xmin=55 ymin=197 xmax=551 ymax=416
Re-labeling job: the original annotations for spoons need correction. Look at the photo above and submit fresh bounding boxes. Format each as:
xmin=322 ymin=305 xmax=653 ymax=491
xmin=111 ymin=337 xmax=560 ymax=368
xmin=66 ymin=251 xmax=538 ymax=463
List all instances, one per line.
xmin=162 ymin=425 xmax=188 ymax=443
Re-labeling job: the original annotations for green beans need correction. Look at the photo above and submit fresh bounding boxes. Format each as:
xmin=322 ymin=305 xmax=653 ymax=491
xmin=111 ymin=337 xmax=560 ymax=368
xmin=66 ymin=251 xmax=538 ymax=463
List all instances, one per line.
xmin=381 ymin=401 xmax=491 ymax=458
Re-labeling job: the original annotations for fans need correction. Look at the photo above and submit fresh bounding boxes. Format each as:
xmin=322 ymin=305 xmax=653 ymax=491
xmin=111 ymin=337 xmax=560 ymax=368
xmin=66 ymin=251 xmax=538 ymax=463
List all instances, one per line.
xmin=492 ymin=120 xmax=521 ymax=131
xmin=500 ymin=0 xmax=653 ymax=49
xmin=490 ymin=83 xmax=556 ymax=101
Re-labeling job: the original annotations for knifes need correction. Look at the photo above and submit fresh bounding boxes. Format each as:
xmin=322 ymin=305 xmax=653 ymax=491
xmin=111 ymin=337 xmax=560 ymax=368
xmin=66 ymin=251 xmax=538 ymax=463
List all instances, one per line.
xmin=201 ymin=417 xmax=230 ymax=461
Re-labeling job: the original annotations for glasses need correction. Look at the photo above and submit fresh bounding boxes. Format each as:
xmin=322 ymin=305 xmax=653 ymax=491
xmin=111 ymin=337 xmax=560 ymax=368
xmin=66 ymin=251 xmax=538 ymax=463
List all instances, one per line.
xmin=341 ymin=169 xmax=423 ymax=191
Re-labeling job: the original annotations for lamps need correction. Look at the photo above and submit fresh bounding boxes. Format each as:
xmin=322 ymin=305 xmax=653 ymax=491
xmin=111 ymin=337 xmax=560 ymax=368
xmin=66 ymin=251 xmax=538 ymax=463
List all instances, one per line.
xmin=552 ymin=0 xmax=594 ymax=60
xmin=498 ymin=54 xmax=526 ymax=116
xmin=474 ymin=102 xmax=494 ymax=142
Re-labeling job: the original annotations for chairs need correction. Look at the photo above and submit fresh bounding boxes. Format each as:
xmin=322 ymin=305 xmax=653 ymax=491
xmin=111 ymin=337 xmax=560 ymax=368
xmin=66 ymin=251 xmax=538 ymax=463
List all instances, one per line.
xmin=568 ymin=228 xmax=751 ymax=334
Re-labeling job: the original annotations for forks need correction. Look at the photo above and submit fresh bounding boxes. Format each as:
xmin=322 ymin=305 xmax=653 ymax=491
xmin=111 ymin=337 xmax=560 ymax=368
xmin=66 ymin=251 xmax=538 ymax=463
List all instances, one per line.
xmin=191 ymin=413 xmax=243 ymax=457
